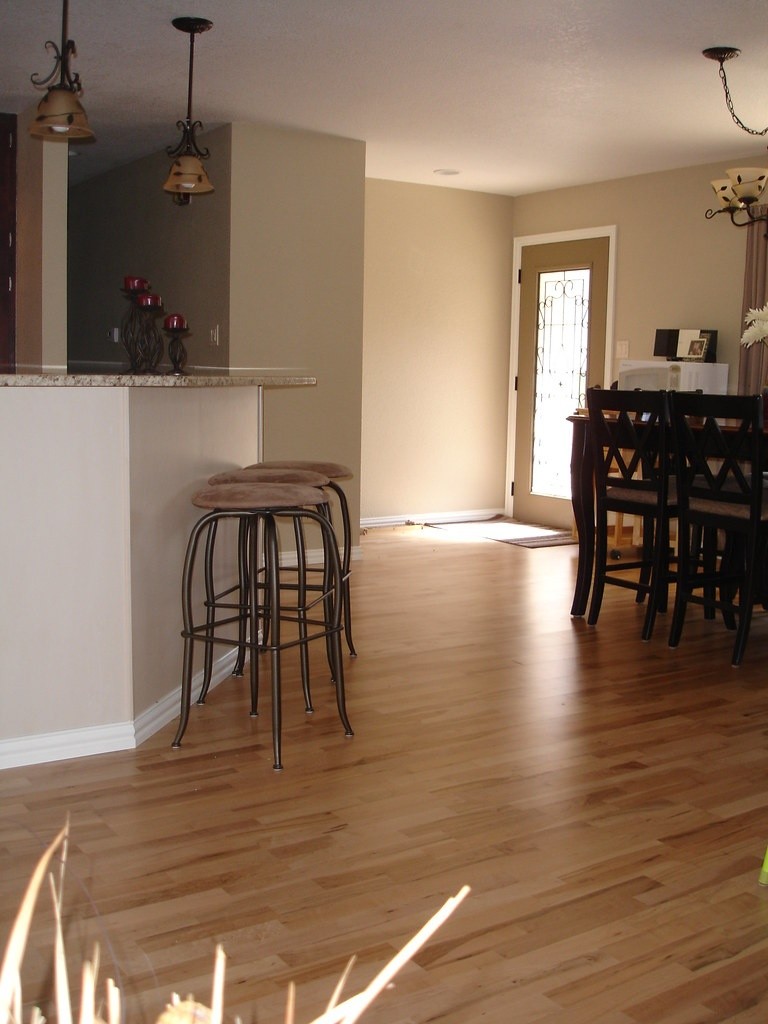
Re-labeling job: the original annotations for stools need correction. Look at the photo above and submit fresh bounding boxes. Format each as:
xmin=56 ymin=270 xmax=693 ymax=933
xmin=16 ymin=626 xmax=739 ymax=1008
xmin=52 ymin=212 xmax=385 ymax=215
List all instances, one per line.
xmin=169 ymin=460 xmax=360 ymax=768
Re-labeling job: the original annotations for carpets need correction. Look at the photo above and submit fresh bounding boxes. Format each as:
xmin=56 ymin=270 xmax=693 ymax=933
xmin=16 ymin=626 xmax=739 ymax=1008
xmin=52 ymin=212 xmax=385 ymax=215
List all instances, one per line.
xmin=424 ymin=514 xmax=579 ymax=548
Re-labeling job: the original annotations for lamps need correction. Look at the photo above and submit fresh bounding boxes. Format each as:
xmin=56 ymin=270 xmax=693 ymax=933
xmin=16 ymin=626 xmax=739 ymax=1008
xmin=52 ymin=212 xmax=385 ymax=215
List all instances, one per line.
xmin=700 ymin=47 xmax=768 ymax=242
xmin=159 ymin=16 xmax=216 ymax=207
xmin=29 ymin=0 xmax=94 ymax=139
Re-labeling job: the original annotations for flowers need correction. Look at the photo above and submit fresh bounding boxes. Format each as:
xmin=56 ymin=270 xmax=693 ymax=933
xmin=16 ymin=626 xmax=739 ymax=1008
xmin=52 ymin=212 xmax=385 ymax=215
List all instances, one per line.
xmin=739 ymin=302 xmax=768 ymax=349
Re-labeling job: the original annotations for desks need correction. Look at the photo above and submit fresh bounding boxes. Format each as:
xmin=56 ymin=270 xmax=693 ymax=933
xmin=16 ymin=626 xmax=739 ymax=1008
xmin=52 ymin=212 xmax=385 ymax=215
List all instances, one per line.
xmin=617 ymin=359 xmax=730 ymax=556
xmin=566 ymin=410 xmax=768 ymax=619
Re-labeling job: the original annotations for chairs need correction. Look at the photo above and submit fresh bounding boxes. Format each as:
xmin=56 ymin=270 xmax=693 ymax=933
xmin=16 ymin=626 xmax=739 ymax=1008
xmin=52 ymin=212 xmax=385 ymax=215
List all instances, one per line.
xmin=586 ymin=386 xmax=707 ymax=644
xmin=610 ymin=381 xmax=717 ymax=604
xmin=666 ymin=388 xmax=768 ymax=668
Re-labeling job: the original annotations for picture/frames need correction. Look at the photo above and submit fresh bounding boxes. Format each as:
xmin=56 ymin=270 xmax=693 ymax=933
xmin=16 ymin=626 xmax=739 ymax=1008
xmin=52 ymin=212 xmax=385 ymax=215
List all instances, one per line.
xmin=685 ymin=338 xmax=707 ymax=357
